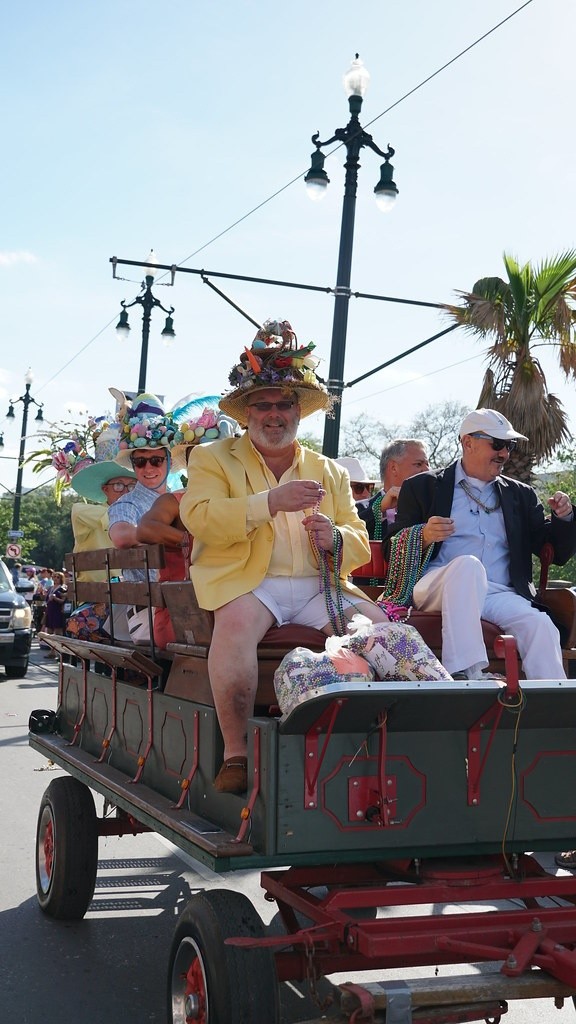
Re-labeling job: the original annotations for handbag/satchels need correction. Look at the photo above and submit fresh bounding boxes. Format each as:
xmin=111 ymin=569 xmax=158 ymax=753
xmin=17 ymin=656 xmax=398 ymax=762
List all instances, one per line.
xmin=41 ymin=613 xmax=48 ymax=625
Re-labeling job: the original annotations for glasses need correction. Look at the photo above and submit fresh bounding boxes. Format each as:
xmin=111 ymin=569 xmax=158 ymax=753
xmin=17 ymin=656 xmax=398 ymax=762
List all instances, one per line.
xmin=348 ymin=483 xmax=375 ymax=494
xmin=105 ymin=482 xmax=136 ymax=493
xmin=131 ymin=456 xmax=168 ymax=468
xmin=469 ymin=434 xmax=517 ymax=453
xmin=247 ymin=401 xmax=297 ymax=412
xmin=54 ymin=576 xmax=59 ymax=579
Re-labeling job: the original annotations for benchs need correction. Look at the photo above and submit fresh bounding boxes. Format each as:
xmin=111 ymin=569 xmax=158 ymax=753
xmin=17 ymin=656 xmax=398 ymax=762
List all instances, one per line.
xmin=41 ymin=543 xmax=575 ymax=712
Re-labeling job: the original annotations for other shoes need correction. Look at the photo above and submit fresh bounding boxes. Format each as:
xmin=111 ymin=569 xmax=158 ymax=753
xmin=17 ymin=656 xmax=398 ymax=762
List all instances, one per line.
xmin=214 ymin=756 xmax=248 ymax=793
xmin=54 ymin=654 xmax=60 ymax=661
xmin=44 ymin=653 xmax=56 ymax=658
xmin=451 ymin=672 xmax=469 ymax=680
xmin=555 ymin=851 xmax=576 ymax=869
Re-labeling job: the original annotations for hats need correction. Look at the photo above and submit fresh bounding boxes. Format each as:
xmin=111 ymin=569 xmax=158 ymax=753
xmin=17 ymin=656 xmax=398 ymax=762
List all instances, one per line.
xmin=218 ymin=317 xmax=342 ymax=427
xmin=17 ymin=411 xmax=139 ymax=506
xmin=25 ymin=567 xmax=36 ymax=573
xmin=107 ymin=388 xmax=184 ymax=474
xmin=170 ymin=393 xmax=242 ymax=469
xmin=459 ymin=408 xmax=529 ymax=442
xmin=330 ymin=456 xmax=375 ymax=484
xmin=14 ymin=563 xmax=21 ymax=566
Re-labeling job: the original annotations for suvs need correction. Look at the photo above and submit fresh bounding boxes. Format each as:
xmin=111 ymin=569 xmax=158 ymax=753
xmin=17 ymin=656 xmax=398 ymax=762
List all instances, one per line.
xmin=0 ymin=558 xmax=34 ymax=679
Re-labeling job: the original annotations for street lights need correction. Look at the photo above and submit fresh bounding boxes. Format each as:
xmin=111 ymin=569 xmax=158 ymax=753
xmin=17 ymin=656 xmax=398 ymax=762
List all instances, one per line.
xmin=114 ymin=247 xmax=176 ymax=398
xmin=300 ymin=49 xmax=399 ymax=463
xmin=0 ymin=364 xmax=45 ymax=567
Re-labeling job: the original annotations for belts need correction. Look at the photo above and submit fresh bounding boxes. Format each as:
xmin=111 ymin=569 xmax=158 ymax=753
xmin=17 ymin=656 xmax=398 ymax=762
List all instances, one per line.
xmin=126 ymin=605 xmax=148 ymax=620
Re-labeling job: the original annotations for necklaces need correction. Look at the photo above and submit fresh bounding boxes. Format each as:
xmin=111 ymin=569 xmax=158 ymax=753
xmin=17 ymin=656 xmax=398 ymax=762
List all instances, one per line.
xmin=370 ymin=494 xmax=387 ymax=586
xmin=308 ymin=481 xmax=362 ymax=637
xmin=376 ymin=523 xmax=434 ymax=623
xmin=460 ymin=481 xmax=502 ymax=512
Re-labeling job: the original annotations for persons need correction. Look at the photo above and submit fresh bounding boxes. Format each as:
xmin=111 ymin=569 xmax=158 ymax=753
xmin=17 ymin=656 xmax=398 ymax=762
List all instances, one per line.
xmin=333 ymin=456 xmax=381 ymax=502
xmin=18 ymin=414 xmax=138 ymax=643
xmin=352 ymin=439 xmax=430 ymax=585
xmin=107 ymin=387 xmax=177 ymax=642
xmin=380 ymin=410 xmax=576 ymax=678
xmin=134 ymin=395 xmax=240 ymax=649
xmin=14 ymin=564 xmax=73 ymax=661
xmin=180 ymin=319 xmax=390 ymax=795
